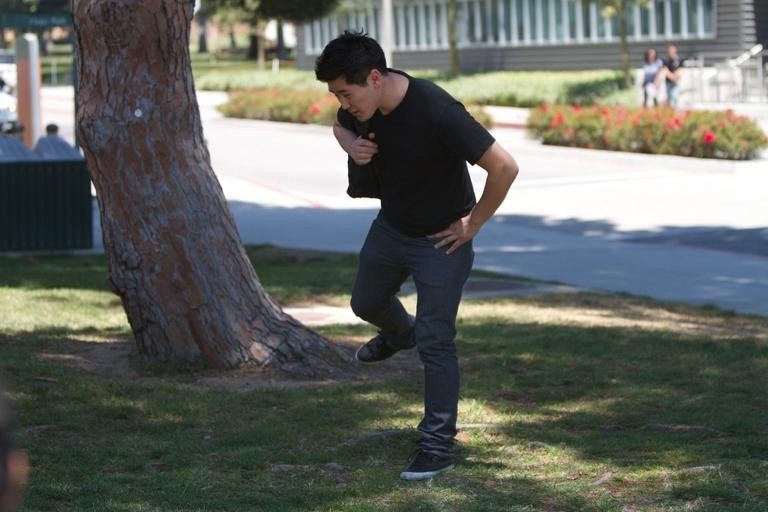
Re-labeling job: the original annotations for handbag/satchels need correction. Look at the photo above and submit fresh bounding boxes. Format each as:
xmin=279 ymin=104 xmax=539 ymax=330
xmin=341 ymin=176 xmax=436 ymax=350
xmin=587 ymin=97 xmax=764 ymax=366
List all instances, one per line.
xmin=346 ymin=141 xmax=383 ymax=200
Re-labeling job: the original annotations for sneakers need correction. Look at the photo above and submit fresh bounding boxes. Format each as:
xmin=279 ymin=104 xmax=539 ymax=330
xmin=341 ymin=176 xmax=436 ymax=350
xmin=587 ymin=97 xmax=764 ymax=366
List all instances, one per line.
xmin=400 ymin=450 xmax=453 ymax=480
xmin=355 ymin=332 xmax=417 ymax=362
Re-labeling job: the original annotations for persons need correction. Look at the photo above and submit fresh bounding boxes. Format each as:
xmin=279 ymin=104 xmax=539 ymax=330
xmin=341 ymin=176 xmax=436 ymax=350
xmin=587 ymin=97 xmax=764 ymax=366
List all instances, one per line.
xmin=641 ymin=43 xmax=684 ymax=107
xmin=315 ymin=29 xmax=518 ymax=482
